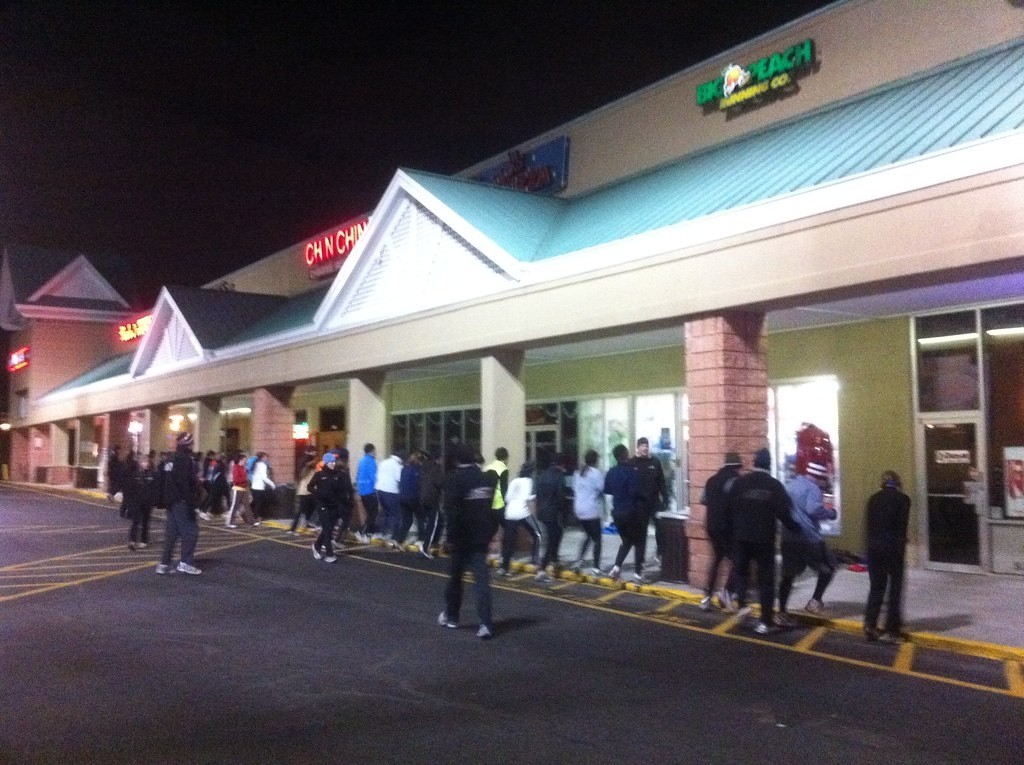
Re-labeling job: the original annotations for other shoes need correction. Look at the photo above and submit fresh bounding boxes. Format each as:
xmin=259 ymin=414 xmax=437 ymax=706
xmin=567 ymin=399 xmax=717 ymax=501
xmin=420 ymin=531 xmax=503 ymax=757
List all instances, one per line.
xmin=477 ymin=624 xmax=492 ymax=639
xmin=498 ymin=560 xmax=650 ymax=586
xmin=877 ymin=632 xmax=910 ymax=646
xmin=754 ymin=622 xmax=781 ymax=635
xmin=196 ymin=510 xmax=262 ymax=529
xmin=437 ymin=612 xmax=459 ymax=627
xmin=715 ymin=587 xmax=738 ymax=614
xmin=805 ymin=599 xmax=831 ymax=615
xmin=129 ymin=540 xmax=137 ymax=551
xmin=774 ymin=611 xmax=801 ymax=628
xmin=135 ymin=541 xmax=147 ymax=548
xmin=864 ymin=627 xmax=879 ymax=641
xmin=287 ymin=523 xmax=440 ymax=562
xmin=700 ymin=597 xmax=720 ymax=613
xmin=738 ymin=607 xmax=756 ymax=629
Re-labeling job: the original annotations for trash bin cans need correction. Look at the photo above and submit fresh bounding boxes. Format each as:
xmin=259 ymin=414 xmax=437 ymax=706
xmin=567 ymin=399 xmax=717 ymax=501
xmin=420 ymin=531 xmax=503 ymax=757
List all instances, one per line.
xmin=653 ymin=513 xmax=690 ymax=583
xmin=78 ymin=467 xmax=99 ymax=489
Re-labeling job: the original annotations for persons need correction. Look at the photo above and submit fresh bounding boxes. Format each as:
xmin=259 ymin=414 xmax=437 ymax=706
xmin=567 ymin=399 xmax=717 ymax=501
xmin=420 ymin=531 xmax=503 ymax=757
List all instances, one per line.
xmin=375 ymin=448 xmax=405 ymax=542
xmin=156 ymin=432 xmax=202 ymax=575
xmin=437 ymin=444 xmax=493 ymax=637
xmin=537 ymin=451 xmax=569 ymax=581
xmin=572 ymin=449 xmax=609 ymax=575
xmin=106 ymin=444 xmax=171 ymax=549
xmin=392 ymin=451 xmax=425 ymax=551
xmin=700 ymin=451 xmax=743 ymax=613
xmin=604 ymin=436 xmax=669 ymax=578
xmin=420 ymin=448 xmax=444 ymax=560
xmin=775 ymin=461 xmax=838 ymax=629
xmin=862 ymin=471 xmax=912 ymax=644
xmin=195 ymin=450 xmax=275 ymax=528
xmin=728 ymin=446 xmax=802 ymax=634
xmin=497 ymin=462 xmax=541 ymax=577
xmin=300 ymin=444 xmax=355 ymax=562
xmin=481 ymin=446 xmax=511 ymax=539
xmin=355 ymin=443 xmax=378 ymax=545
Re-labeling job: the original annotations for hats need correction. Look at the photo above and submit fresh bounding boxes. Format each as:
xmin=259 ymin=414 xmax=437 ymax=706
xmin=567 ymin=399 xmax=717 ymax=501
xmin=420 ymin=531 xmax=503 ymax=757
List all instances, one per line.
xmin=175 ymin=431 xmax=194 ymax=446
xmin=724 ymin=450 xmax=743 ymax=466
xmin=807 ymin=460 xmax=829 ymax=479
xmin=879 ymin=470 xmax=902 ymax=487
xmin=521 ymin=461 xmax=536 ymax=472
xmin=754 ymin=447 xmax=771 ymax=467
xmin=323 ymin=452 xmax=336 ymax=464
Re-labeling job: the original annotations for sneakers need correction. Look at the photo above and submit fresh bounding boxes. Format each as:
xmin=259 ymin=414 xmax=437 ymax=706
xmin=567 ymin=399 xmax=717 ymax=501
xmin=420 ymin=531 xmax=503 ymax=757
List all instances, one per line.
xmin=155 ymin=564 xmax=170 ymax=575
xmin=176 ymin=562 xmax=201 ymax=575
